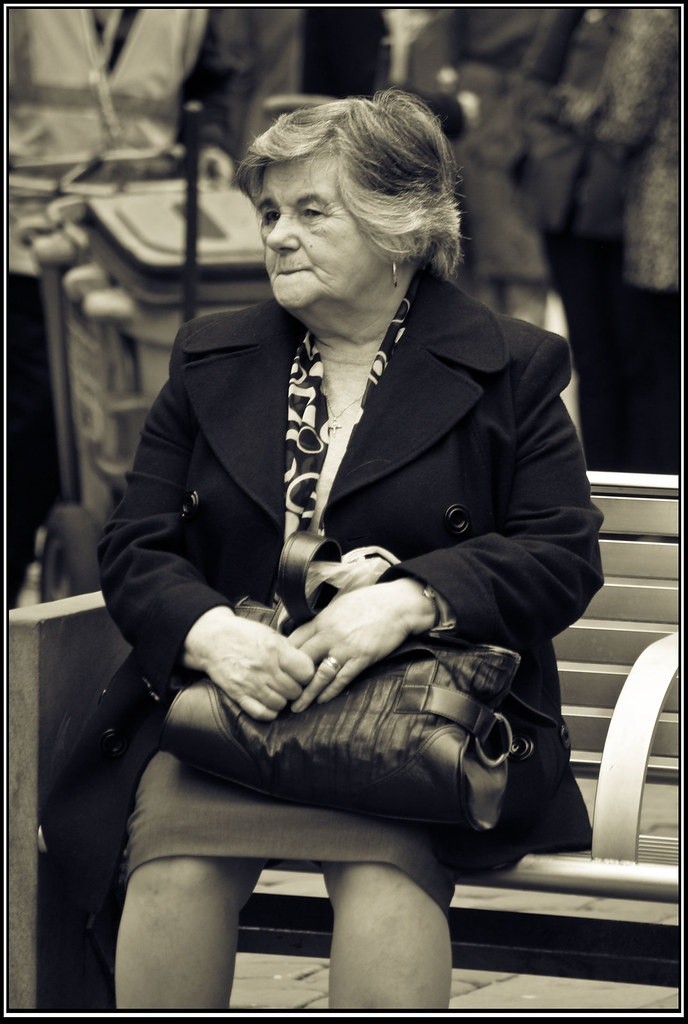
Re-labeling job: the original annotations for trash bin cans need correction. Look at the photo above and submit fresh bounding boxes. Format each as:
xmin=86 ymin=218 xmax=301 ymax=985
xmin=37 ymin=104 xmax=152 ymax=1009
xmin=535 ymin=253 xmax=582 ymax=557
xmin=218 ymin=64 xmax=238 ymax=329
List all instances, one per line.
xmin=31 ymin=170 xmax=278 ymax=583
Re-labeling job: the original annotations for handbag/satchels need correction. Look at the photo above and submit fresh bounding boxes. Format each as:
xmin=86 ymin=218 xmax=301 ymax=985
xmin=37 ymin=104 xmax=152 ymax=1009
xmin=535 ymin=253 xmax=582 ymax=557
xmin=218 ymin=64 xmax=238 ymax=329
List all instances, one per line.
xmin=156 ymin=533 xmax=513 ymax=834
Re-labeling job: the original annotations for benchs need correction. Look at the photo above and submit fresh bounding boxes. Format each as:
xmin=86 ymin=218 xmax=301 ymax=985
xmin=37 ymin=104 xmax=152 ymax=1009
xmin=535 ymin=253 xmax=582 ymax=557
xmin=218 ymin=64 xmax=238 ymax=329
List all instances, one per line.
xmin=9 ymin=470 xmax=680 ymax=1011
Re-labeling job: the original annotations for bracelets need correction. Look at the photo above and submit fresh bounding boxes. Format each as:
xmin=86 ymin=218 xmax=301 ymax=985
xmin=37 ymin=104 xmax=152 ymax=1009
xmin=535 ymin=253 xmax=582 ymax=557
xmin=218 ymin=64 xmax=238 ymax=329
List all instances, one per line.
xmin=416 ymin=575 xmax=441 ymax=627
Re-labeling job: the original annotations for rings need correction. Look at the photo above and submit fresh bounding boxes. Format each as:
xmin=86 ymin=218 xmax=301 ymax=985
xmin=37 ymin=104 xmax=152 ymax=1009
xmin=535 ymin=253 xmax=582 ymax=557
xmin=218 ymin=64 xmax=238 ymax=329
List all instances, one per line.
xmin=323 ymin=656 xmax=341 ymax=671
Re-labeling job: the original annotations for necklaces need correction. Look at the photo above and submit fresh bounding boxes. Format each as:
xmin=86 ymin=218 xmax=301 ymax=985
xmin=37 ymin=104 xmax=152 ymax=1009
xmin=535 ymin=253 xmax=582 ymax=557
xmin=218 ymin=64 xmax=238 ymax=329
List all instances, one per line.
xmin=322 ymin=381 xmax=363 ymax=433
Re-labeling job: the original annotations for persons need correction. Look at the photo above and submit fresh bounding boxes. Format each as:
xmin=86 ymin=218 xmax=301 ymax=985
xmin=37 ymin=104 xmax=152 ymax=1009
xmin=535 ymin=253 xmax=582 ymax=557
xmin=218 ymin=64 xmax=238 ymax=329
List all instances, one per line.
xmin=96 ymin=90 xmax=593 ymax=1007
xmin=6 ymin=6 xmax=679 ymax=607
